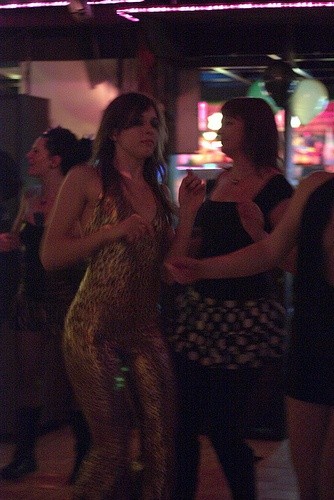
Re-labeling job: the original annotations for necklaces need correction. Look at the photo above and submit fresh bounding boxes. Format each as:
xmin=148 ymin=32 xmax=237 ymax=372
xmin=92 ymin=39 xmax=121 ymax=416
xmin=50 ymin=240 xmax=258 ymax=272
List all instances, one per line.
xmin=40 ymin=199 xmax=47 ymax=204
xmin=232 ymin=179 xmax=239 ymax=184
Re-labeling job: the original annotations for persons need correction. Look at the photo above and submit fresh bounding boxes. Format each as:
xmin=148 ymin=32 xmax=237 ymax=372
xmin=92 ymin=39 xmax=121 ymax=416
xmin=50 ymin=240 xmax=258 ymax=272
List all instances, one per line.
xmin=161 ymin=170 xmax=334 ymax=500
xmin=0 ymin=150 xmax=25 ymax=313
xmin=0 ymin=127 xmax=96 ymax=486
xmin=40 ymin=91 xmax=207 ymax=500
xmin=162 ymin=95 xmax=287 ymax=500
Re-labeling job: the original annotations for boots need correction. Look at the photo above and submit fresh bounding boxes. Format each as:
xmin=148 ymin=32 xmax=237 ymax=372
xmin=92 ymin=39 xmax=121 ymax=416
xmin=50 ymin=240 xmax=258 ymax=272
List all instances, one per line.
xmin=0 ymin=406 xmax=41 ymax=483
xmin=65 ymin=418 xmax=95 ymax=485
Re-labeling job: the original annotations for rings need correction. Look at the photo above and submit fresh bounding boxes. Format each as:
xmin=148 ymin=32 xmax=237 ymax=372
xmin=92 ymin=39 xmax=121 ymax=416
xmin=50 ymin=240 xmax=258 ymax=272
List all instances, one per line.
xmin=139 ymin=227 xmax=142 ymax=231
